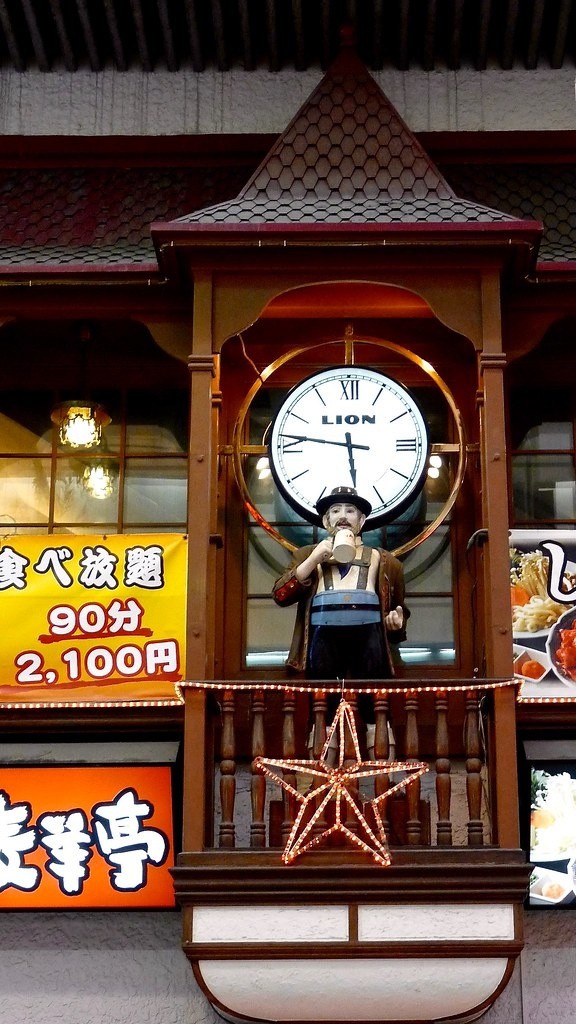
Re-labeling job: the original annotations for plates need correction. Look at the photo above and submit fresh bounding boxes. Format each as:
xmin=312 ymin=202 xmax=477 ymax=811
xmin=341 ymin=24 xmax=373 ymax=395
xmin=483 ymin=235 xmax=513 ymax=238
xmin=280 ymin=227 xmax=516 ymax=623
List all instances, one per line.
xmin=528 ymin=866 xmax=576 ymax=906
xmin=513 ymin=559 xmax=576 ymax=639
xmin=512 ymin=643 xmax=551 ymax=684
xmin=546 ymin=605 xmax=576 ymax=690
xmin=400 ymin=646 xmax=432 ymax=663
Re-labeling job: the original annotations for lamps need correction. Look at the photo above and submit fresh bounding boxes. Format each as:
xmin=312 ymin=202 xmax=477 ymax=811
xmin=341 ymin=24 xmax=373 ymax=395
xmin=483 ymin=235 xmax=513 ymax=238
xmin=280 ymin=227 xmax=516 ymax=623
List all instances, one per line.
xmin=78 ymin=460 xmax=118 ymax=498
xmin=51 ymin=394 xmax=112 ymax=450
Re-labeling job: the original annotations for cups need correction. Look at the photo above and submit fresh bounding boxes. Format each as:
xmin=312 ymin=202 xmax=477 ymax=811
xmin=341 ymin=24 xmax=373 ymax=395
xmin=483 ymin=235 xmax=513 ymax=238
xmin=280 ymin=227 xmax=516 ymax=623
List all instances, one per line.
xmin=332 ymin=529 xmax=357 ymax=564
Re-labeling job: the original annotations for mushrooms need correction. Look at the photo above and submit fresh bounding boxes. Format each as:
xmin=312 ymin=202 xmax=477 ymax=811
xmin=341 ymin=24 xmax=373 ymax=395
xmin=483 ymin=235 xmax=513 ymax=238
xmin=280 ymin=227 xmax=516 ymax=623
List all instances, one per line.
xmin=509 ymin=550 xmax=576 ymax=635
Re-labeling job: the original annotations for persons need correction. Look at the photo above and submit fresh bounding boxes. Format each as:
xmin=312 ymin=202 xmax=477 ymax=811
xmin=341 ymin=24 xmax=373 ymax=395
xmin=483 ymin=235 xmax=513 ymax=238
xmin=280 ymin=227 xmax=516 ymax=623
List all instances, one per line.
xmin=272 ymin=487 xmax=411 ymax=680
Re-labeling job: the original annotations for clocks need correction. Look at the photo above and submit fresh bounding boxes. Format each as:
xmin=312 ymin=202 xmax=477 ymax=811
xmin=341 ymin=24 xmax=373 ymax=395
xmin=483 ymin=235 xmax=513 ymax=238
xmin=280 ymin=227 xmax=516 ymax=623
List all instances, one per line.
xmin=269 ymin=367 xmax=432 ymax=532
